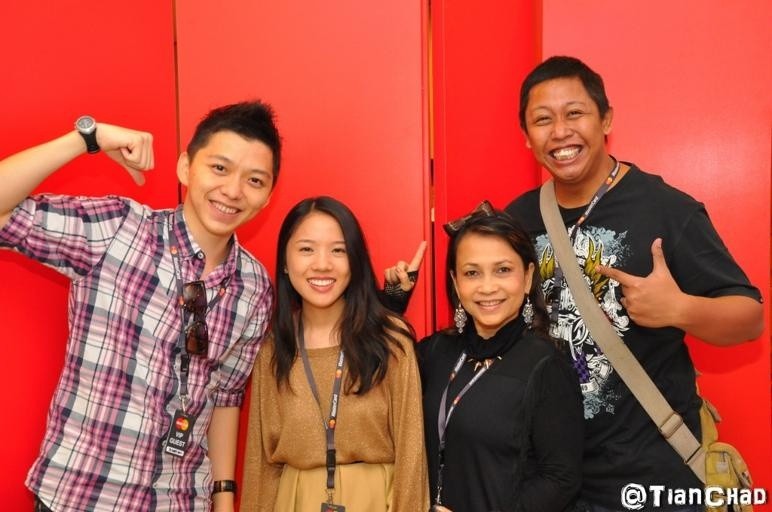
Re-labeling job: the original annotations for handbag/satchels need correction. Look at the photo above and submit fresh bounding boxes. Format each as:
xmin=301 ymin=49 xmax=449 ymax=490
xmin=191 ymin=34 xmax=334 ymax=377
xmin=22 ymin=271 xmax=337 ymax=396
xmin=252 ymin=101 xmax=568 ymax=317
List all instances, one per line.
xmin=706 ymin=440 xmax=754 ymax=512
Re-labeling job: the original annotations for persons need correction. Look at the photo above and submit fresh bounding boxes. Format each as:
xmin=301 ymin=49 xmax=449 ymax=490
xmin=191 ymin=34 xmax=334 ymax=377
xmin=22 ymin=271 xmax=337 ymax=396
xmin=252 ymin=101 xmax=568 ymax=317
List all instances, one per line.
xmin=0 ymin=97 xmax=286 ymax=512
xmin=503 ymin=54 xmax=766 ymax=510
xmin=381 ymin=200 xmax=586 ymax=511
xmin=239 ymin=193 xmax=433 ymax=511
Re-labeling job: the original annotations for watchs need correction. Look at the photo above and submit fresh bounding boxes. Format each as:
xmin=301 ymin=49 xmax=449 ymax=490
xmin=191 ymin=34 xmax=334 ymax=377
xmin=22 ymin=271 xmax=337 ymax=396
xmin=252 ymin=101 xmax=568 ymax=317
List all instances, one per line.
xmin=74 ymin=113 xmax=99 ymax=155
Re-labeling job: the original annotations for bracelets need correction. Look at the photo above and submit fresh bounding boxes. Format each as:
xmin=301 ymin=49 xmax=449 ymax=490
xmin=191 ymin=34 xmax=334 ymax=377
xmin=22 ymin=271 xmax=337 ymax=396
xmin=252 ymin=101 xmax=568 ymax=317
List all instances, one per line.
xmin=211 ymin=480 xmax=237 ymax=493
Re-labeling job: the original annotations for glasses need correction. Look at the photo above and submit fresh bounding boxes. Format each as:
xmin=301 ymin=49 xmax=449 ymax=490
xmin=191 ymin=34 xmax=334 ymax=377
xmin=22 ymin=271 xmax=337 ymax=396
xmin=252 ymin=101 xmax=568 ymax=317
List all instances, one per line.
xmin=179 ymin=279 xmax=210 ymax=357
